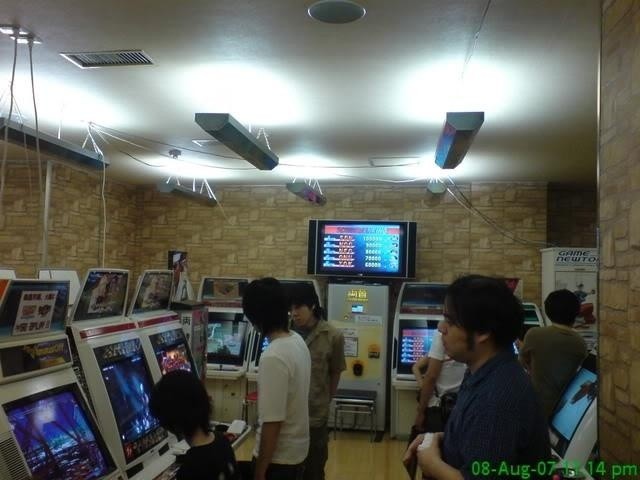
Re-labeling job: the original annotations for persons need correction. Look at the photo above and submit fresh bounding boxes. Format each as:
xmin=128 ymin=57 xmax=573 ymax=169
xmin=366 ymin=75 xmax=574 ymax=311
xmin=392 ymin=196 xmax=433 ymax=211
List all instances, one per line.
xmin=516 ymin=287 xmax=591 ymax=428
xmin=400 ymin=275 xmax=554 ymax=479
xmin=238 ymin=277 xmax=315 ymax=479
xmin=409 ymin=352 xmax=431 ymax=389
xmin=146 ymin=367 xmax=245 ymax=480
xmin=513 ymin=324 xmax=530 ymax=349
xmin=284 ymin=280 xmax=349 ymax=480
xmin=402 ymin=331 xmax=469 ymax=434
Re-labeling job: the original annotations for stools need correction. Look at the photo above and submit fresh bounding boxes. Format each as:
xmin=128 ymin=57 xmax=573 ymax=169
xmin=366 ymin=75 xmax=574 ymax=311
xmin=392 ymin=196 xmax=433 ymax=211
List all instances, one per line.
xmin=241 ymin=389 xmax=257 ymax=422
xmin=334 ymin=394 xmax=377 ymax=441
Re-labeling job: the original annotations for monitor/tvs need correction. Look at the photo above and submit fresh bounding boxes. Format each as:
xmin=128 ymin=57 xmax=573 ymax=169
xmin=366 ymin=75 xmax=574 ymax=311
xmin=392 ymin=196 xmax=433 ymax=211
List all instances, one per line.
xmin=546 ymin=352 xmax=597 ymax=459
xmin=397 ymin=326 xmax=443 ymax=374
xmin=100 ymin=350 xmax=161 ymax=448
xmin=2 ymin=381 xmax=119 ymax=480
xmin=254 ymin=334 xmax=271 ymax=367
xmin=305 ymin=220 xmax=416 ymax=285
xmin=205 ymin=318 xmax=249 ymax=366
xmin=156 ymin=338 xmax=201 ymax=384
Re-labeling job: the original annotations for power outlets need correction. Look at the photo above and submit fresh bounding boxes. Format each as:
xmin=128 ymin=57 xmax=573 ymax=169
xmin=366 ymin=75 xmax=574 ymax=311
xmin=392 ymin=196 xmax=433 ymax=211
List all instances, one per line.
xmin=0 ymin=25 xmax=41 ymax=45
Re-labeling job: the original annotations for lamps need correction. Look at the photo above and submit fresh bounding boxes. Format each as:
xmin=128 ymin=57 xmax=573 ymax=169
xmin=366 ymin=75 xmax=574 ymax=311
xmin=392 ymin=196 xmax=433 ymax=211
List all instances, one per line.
xmin=0 ymin=116 xmax=110 ymax=170
xmin=157 ymin=172 xmax=217 ymax=208
xmin=194 ymin=113 xmax=278 ymax=171
xmin=436 ymin=111 xmax=484 ymax=170
xmin=286 ymin=178 xmax=326 ymax=206
xmin=425 ymin=178 xmax=445 ymax=197
